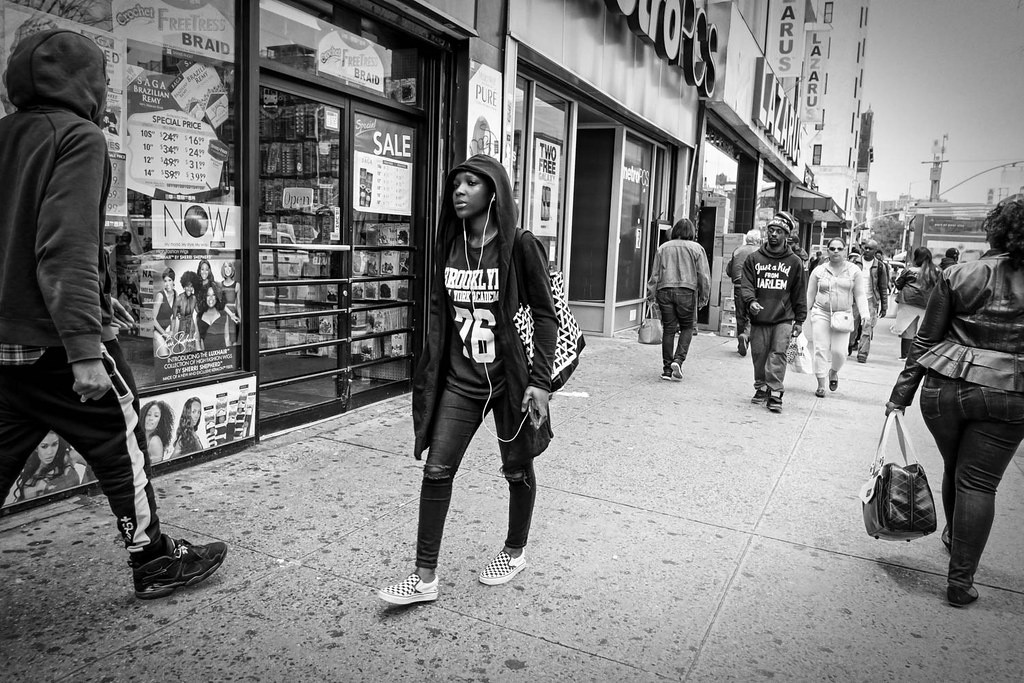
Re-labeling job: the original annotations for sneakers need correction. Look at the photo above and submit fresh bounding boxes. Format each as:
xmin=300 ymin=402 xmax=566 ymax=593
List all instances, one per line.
xmin=767 ymin=388 xmax=782 ymax=410
xmin=751 ymin=385 xmax=767 ymax=403
xmin=127 ymin=534 xmax=227 ymax=599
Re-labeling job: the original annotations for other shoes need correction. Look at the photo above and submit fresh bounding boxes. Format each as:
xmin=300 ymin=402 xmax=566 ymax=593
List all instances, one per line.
xmin=816 ymin=388 xmax=825 ymax=397
xmin=738 ymin=333 xmax=749 ymax=356
xmin=897 ymin=356 xmax=908 ymax=361
xmin=946 ymin=585 xmax=979 ymax=608
xmin=670 ymin=361 xmax=683 ymax=380
xmin=829 ymin=369 xmax=839 ymax=391
xmin=941 ymin=524 xmax=953 ymax=552
xmin=661 ymin=372 xmax=671 ymax=380
xmin=478 ymin=549 xmax=528 ymax=585
xmin=380 ymin=573 xmax=440 ymax=603
xmin=858 ymin=354 xmax=865 ymax=362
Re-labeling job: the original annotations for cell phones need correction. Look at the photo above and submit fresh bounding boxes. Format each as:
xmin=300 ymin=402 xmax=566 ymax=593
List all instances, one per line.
xmin=528 ymin=398 xmax=539 ymax=429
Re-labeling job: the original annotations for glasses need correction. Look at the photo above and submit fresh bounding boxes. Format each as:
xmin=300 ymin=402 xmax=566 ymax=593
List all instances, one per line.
xmin=829 ymin=246 xmax=842 ymax=251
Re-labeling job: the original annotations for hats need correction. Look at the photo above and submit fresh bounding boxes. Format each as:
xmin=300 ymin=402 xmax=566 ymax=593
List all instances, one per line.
xmin=768 ymin=210 xmax=794 ymax=235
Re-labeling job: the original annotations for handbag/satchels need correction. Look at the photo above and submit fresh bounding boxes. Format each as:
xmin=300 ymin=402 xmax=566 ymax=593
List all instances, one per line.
xmin=511 ymin=228 xmax=586 ymax=392
xmin=830 ymin=310 xmax=854 ymax=333
xmin=638 ymin=303 xmax=662 ymax=344
xmin=859 ymin=408 xmax=936 ymax=544
xmin=786 ymin=328 xmax=812 ymax=375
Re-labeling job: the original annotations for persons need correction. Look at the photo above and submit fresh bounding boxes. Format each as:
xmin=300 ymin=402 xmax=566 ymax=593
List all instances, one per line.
xmin=171 ymin=397 xmax=203 ymax=459
xmin=740 ymin=211 xmax=807 ymax=412
xmin=806 ymin=238 xmax=871 ymax=397
xmin=377 ymin=154 xmax=559 ymax=605
xmin=645 ymin=218 xmax=711 ymax=382
xmin=141 ymin=400 xmax=176 ymax=464
xmin=152 ymin=260 xmax=241 ymax=358
xmin=725 ymin=229 xmax=960 ymax=363
xmin=13 ymin=430 xmax=96 ymax=503
xmin=0 ymin=28 xmax=227 ymax=600
xmin=884 ymin=200 xmax=1024 ymax=608
xmin=115 ymin=231 xmax=140 ymax=321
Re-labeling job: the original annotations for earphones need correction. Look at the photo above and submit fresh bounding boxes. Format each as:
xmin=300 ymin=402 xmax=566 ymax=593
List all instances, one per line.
xmin=490 ymin=192 xmax=496 ymax=203
